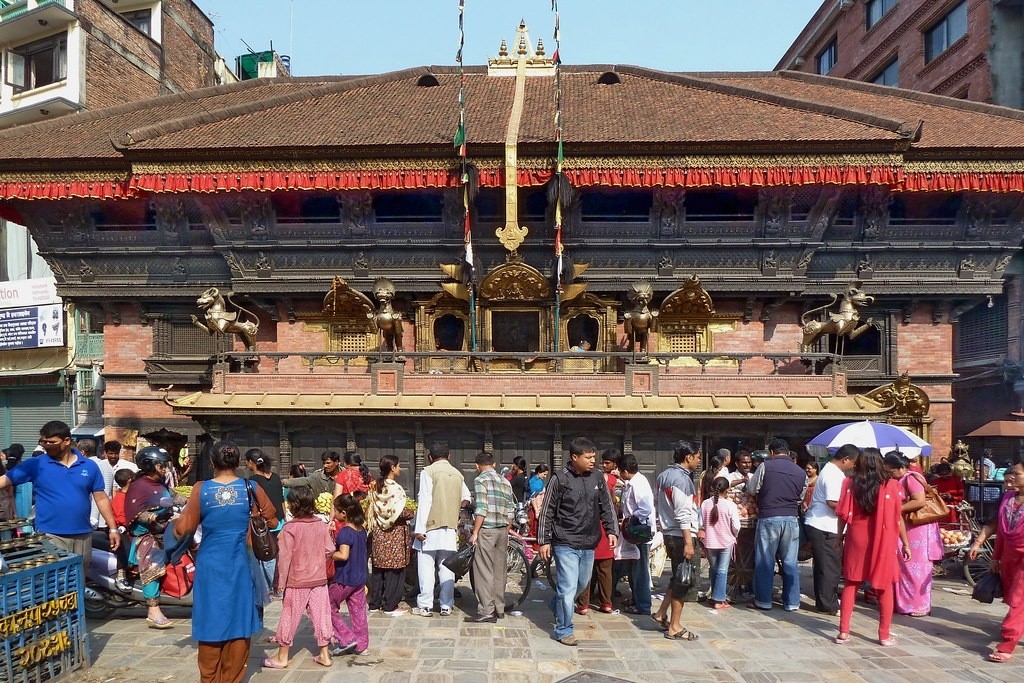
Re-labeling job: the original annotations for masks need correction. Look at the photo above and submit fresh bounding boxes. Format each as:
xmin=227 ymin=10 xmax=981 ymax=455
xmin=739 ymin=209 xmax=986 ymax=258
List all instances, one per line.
xmin=40 ymin=439 xmax=68 ymax=458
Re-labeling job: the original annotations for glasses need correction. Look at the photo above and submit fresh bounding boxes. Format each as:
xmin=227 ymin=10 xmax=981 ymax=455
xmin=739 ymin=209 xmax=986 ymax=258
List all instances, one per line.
xmin=156 ymin=463 xmax=167 ymax=468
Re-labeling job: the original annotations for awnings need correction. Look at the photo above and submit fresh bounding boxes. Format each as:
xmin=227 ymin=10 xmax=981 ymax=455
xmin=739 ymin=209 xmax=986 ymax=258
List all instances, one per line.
xmin=966 ymin=419 xmax=1024 ymax=437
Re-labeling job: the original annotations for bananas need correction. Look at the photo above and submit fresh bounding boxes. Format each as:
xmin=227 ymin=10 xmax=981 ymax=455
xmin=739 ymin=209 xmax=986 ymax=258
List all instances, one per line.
xmin=314 ymin=495 xmax=333 ymax=514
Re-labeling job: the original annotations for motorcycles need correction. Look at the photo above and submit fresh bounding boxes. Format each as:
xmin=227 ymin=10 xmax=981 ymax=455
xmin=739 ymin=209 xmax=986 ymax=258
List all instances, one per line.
xmin=84 ymin=497 xmax=204 ymax=620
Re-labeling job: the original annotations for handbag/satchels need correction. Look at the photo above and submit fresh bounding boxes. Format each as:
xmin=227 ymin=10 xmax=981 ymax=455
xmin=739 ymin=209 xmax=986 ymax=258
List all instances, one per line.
xmin=675 ymin=557 xmax=696 ymax=587
xmin=622 ymin=515 xmax=652 ymax=544
xmin=160 ymin=555 xmax=195 ymax=599
xmin=972 ymin=571 xmax=1004 ymax=604
xmin=442 ymin=543 xmax=475 ymax=577
xmin=904 ymin=473 xmax=950 ymax=523
xmin=242 ymin=476 xmax=277 ymax=561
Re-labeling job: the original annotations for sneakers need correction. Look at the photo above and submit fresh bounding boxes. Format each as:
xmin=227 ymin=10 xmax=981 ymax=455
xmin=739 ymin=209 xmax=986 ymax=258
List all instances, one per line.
xmin=411 ymin=605 xmax=454 ymax=617
xmin=116 ymin=577 xmax=132 ymax=592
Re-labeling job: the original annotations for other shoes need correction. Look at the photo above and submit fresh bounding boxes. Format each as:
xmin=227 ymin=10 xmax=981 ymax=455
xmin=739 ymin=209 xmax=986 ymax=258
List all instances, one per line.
xmin=707 ymin=595 xmax=844 ymax=616
xmin=575 ymin=600 xmax=652 ymax=615
xmin=556 ymin=634 xmax=579 ymax=646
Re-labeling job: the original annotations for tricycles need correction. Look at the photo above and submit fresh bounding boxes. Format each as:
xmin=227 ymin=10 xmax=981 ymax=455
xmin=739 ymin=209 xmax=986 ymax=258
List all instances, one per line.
xmin=837 ymin=499 xmax=997 ymax=587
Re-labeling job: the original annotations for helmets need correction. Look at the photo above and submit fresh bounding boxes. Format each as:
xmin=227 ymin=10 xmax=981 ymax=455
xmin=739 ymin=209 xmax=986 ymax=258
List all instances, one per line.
xmin=750 ymin=449 xmax=772 ymax=467
xmin=136 ymin=446 xmax=172 ymax=472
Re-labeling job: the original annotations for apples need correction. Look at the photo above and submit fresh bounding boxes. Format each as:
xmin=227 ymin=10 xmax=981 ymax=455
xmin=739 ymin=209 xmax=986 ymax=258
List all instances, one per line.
xmin=938 ymin=527 xmax=965 ymax=544
xmin=733 ymin=491 xmax=760 ymax=518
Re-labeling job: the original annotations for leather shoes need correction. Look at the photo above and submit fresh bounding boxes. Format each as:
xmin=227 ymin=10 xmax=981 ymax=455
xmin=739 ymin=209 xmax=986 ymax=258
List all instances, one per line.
xmin=464 ymin=610 xmax=506 ymax=622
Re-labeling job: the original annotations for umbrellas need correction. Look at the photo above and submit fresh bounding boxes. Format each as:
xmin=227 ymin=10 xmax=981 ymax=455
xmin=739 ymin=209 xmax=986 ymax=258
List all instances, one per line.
xmin=805 ymin=418 xmax=932 ymax=460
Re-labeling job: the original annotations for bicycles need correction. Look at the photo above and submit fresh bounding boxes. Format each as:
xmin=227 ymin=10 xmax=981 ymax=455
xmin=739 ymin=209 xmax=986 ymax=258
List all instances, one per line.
xmin=456 ymin=504 xmax=597 ymax=613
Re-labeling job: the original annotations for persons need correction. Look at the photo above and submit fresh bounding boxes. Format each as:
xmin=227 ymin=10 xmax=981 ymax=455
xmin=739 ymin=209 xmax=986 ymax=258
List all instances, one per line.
xmin=647 ymin=437 xmax=1024 ymax=664
xmin=0 ymin=420 xmax=473 ymax=683
xmin=576 ymin=340 xmax=591 ymax=352
xmin=464 ymin=436 xmax=658 ymax=645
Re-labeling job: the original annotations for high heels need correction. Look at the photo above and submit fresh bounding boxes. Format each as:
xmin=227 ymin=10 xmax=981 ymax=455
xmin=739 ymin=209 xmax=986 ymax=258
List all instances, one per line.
xmin=146 ymin=615 xmax=173 ymax=628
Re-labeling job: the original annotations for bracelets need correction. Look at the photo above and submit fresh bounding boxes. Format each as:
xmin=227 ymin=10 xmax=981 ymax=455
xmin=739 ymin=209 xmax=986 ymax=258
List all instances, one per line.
xmin=109 ymin=528 xmax=122 ymax=534
xmin=974 ymin=539 xmax=983 ymax=548
xmin=901 ymin=545 xmax=911 ymax=549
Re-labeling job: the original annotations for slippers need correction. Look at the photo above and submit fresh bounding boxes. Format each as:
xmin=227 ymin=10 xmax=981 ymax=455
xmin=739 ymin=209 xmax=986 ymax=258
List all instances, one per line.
xmin=312 ymin=654 xmax=333 ymax=667
xmin=260 ymin=656 xmax=286 ymax=669
xmin=881 ymin=636 xmax=898 ymax=646
xmin=835 ymin=633 xmax=851 ymax=643
xmin=649 ymin=612 xmax=700 ymax=641
xmin=332 ymin=641 xmax=371 ymax=656
xmin=986 ymin=651 xmax=1014 ymax=663
